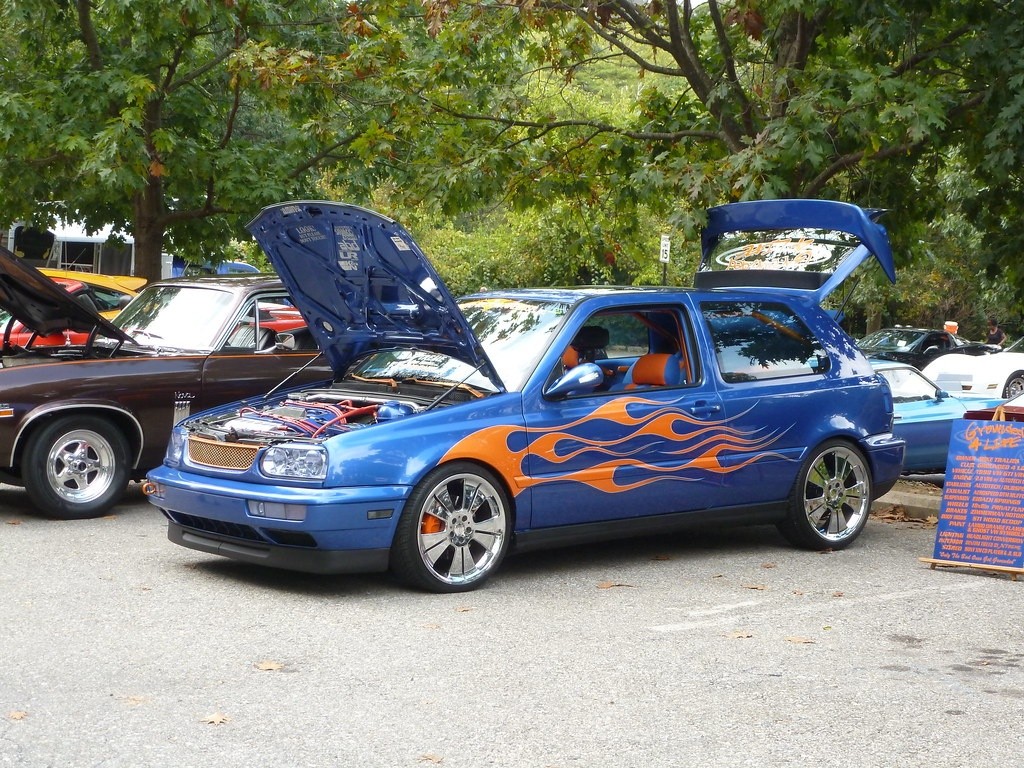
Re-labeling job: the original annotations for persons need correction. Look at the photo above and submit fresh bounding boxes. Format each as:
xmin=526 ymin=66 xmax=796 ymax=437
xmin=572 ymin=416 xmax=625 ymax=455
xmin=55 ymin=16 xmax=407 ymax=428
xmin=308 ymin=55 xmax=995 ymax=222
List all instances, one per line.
xmin=982 ymin=316 xmax=1007 ymax=350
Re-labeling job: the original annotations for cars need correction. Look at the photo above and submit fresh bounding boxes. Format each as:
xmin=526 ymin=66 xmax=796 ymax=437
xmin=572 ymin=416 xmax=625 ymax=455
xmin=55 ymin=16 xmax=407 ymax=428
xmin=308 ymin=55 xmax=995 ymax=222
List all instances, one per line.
xmin=0 ymin=277 xmax=137 ymax=351
xmin=0 ymin=243 xmax=371 ymax=522
xmin=804 ymin=358 xmax=1007 ymax=491
xmin=142 ymin=196 xmax=909 ymax=593
xmin=922 ymin=337 xmax=1024 ymax=402
xmin=853 ymin=327 xmax=1002 ymax=373
xmin=963 ymin=390 xmax=1024 ymax=424
xmin=172 ymin=251 xmax=259 ymax=279
xmin=34 ymin=266 xmax=162 ymax=324
